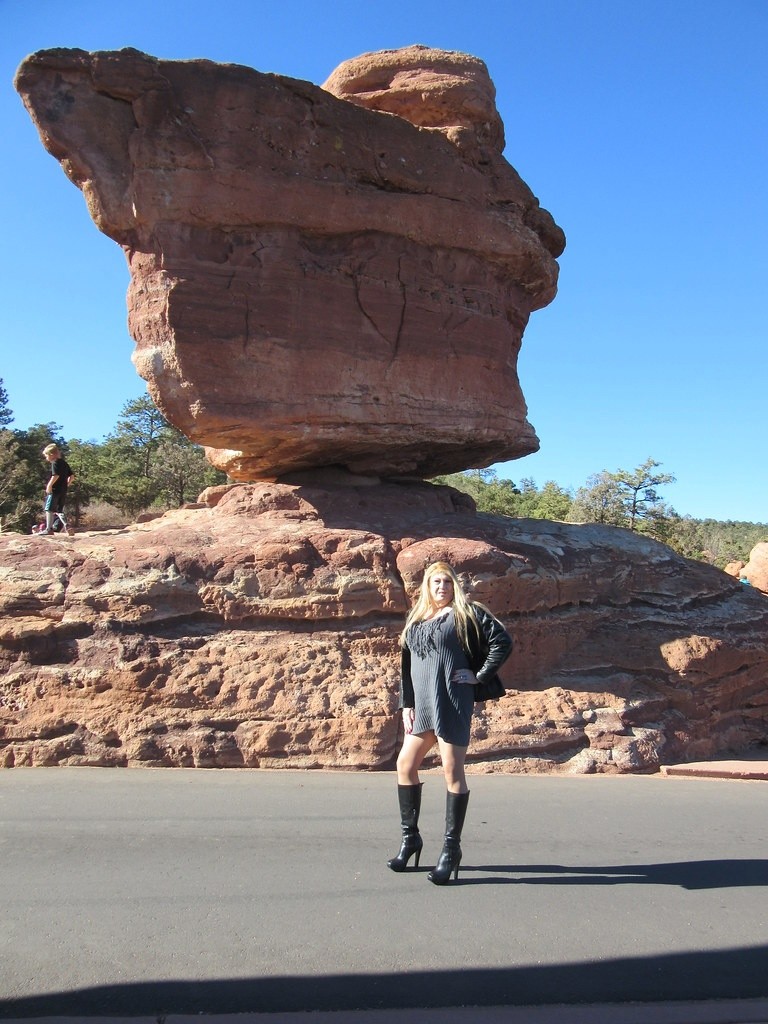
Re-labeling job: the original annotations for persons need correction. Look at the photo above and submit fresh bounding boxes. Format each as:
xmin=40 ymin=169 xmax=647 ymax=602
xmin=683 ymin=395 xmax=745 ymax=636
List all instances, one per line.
xmin=32 ymin=443 xmax=75 ymax=536
xmin=386 ymin=562 xmax=514 ymax=886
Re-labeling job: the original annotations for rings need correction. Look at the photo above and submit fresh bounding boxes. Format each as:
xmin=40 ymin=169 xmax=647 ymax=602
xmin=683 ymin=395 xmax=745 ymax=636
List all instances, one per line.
xmin=461 ymin=676 xmax=462 ymax=680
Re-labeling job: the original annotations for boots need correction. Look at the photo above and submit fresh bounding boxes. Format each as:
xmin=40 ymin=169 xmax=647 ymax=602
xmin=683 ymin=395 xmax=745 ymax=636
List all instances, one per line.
xmin=387 ymin=782 xmax=424 ymax=872
xmin=427 ymin=791 xmax=471 ymax=885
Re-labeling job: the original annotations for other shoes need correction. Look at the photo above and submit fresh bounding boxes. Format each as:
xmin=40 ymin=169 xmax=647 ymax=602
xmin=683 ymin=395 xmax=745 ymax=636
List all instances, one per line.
xmin=65 ymin=523 xmax=74 ymax=536
xmin=39 ymin=528 xmax=54 ymax=535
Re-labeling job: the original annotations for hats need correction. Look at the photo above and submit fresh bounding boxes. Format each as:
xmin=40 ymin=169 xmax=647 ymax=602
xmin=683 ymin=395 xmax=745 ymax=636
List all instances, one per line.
xmin=40 ymin=524 xmax=46 ymax=529
xmin=32 ymin=525 xmax=39 ymax=529
